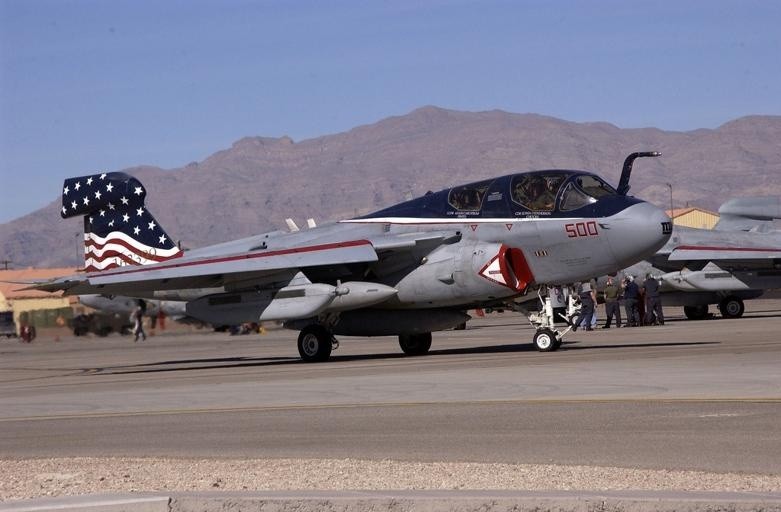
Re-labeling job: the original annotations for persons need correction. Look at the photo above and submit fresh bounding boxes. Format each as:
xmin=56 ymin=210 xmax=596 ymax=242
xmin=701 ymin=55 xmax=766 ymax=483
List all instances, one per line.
xmin=451 ymin=174 xmax=585 ymax=212
xmin=128 ymin=299 xmax=148 ymax=343
xmin=572 ymin=273 xmax=666 ymax=330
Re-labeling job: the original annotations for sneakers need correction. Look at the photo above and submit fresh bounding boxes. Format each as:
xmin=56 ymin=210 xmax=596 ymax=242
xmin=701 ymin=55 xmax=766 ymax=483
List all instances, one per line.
xmin=573 ymin=326 xmax=577 ymax=331
xmin=586 ymin=327 xmax=593 ymax=330
xmin=602 ymin=326 xmax=610 ymax=328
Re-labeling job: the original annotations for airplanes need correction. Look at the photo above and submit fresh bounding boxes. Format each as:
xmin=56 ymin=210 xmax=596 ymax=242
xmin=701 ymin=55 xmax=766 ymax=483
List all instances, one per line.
xmin=488 ymin=193 xmax=781 ymax=321
xmin=1 ymin=149 xmax=677 ymax=364
